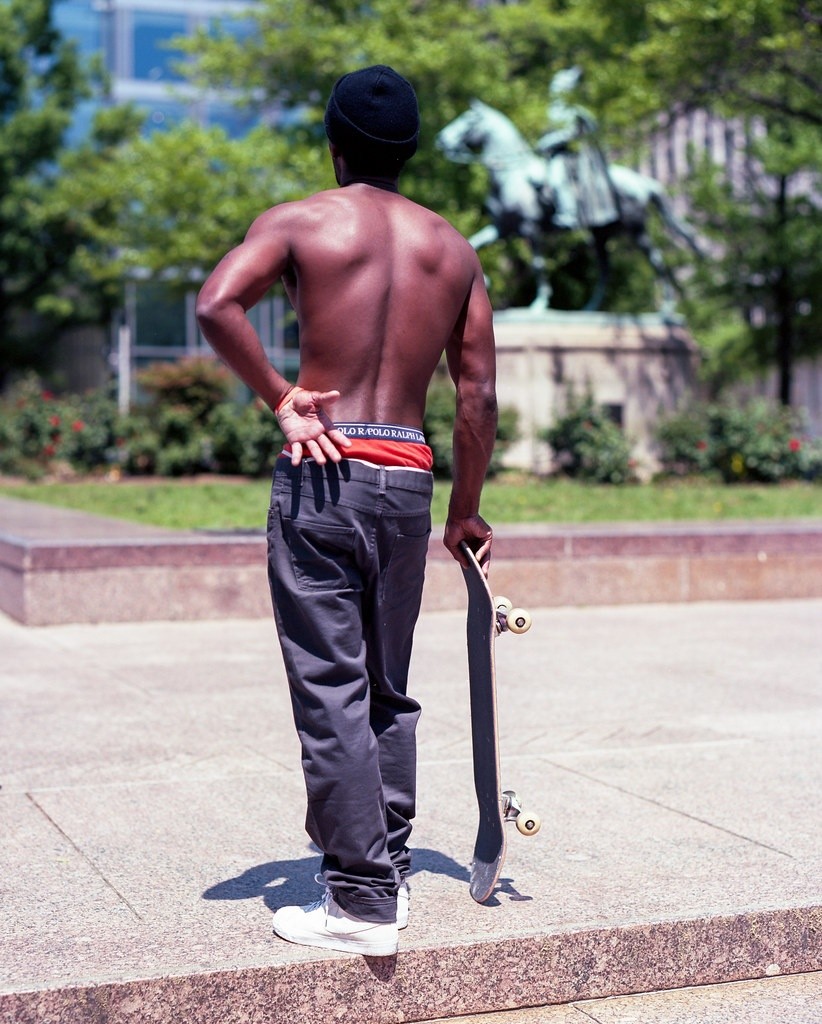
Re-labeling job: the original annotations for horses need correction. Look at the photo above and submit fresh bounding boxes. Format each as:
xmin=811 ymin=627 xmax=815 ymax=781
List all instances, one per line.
xmin=432 ymin=95 xmax=712 ymax=314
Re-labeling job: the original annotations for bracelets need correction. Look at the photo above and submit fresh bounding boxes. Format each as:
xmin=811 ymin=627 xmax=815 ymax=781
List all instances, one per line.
xmin=273 ymin=386 xmax=304 ymax=416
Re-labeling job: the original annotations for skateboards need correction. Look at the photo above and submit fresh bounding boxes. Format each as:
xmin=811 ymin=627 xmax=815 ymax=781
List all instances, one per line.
xmin=455 ymin=538 xmax=541 ymax=903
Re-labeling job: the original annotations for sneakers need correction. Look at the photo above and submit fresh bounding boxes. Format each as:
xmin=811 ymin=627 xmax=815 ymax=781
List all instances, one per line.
xmin=272 ymin=872 xmax=399 ymax=956
xmin=395 ymin=882 xmax=410 ymax=928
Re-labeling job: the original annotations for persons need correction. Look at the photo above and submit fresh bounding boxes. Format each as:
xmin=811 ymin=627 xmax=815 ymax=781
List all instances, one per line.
xmin=535 ymin=64 xmax=621 ymax=227
xmin=194 ymin=62 xmax=500 ymax=959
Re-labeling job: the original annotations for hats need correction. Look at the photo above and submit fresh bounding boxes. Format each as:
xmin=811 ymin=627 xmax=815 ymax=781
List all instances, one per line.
xmin=324 ymin=65 xmax=421 ymax=161
